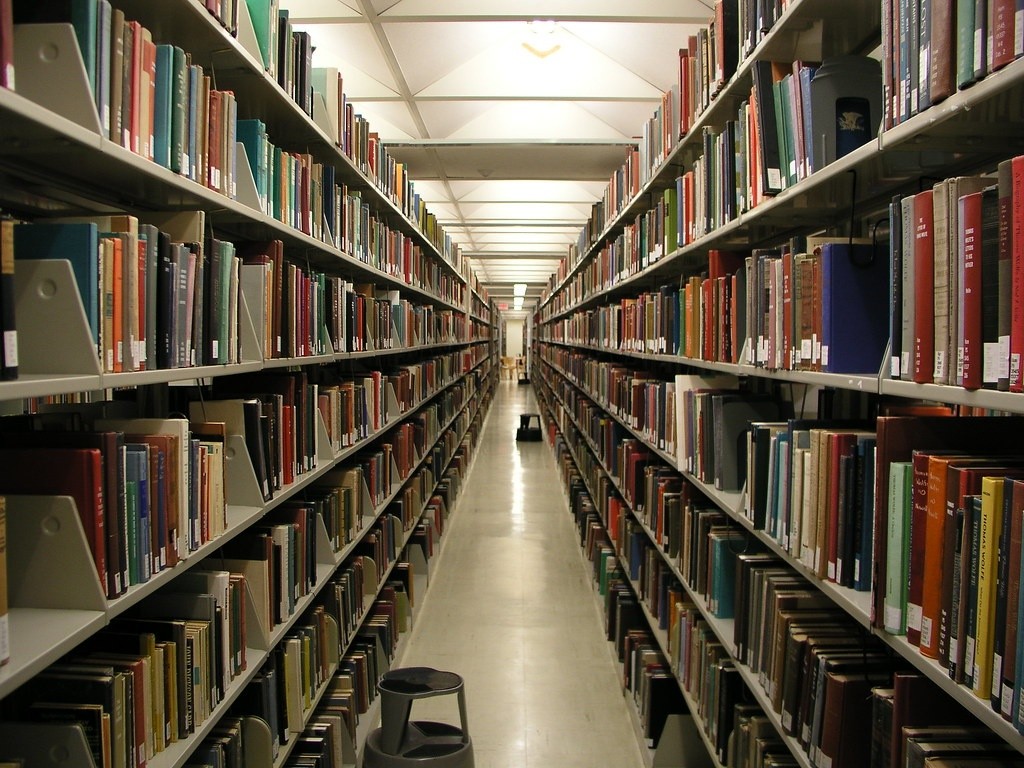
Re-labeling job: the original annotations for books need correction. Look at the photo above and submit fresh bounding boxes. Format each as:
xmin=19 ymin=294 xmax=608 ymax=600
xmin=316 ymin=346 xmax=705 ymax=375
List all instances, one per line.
xmin=0 ymin=1 xmax=500 ymax=767
xmin=521 ymin=0 xmax=1024 ymax=768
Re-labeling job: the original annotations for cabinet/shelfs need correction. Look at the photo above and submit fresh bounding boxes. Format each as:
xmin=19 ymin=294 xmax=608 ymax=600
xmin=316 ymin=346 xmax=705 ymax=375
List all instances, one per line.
xmin=1 ymin=0 xmax=1024 ymax=768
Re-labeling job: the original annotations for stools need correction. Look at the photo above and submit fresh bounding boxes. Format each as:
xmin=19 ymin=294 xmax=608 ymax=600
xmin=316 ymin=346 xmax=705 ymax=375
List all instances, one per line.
xmin=515 ymin=413 xmax=543 ymax=442
xmin=361 ymin=665 xmax=476 ymax=768
xmin=518 ymin=372 xmax=530 ymax=385
